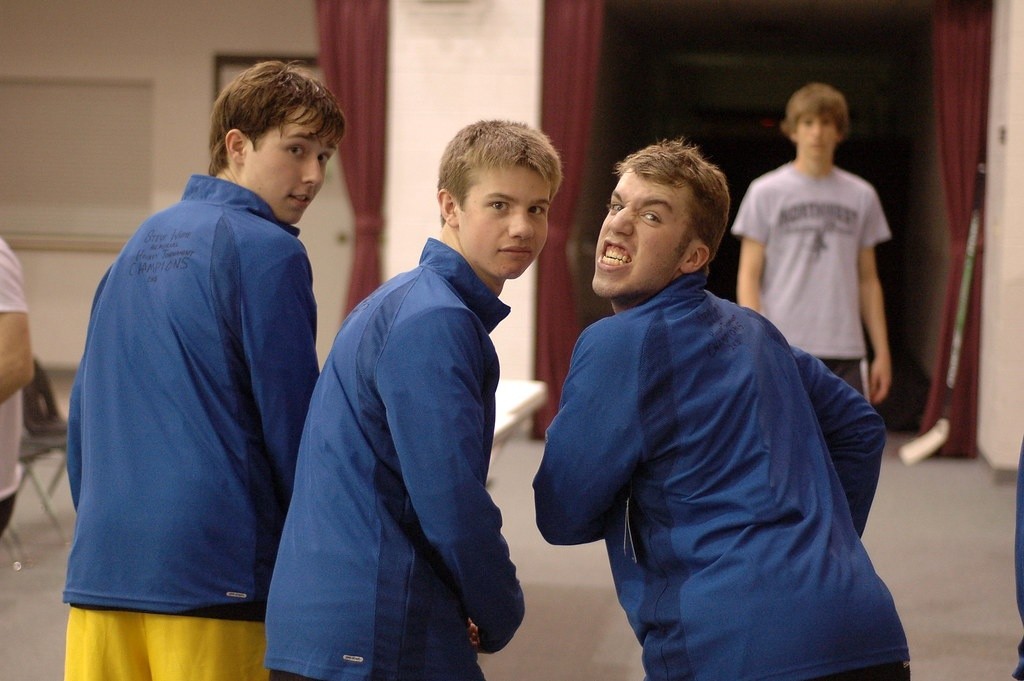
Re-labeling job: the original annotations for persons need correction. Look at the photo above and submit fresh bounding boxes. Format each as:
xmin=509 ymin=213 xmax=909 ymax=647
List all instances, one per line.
xmin=265 ymin=120 xmax=563 ymax=681
xmin=532 ymin=134 xmax=910 ymax=681
xmin=1012 ymin=434 xmax=1024 ymax=681
xmin=0 ymin=237 xmax=35 ymax=538
xmin=731 ymin=82 xmax=891 ymax=408
xmin=61 ymin=61 xmax=346 ymax=681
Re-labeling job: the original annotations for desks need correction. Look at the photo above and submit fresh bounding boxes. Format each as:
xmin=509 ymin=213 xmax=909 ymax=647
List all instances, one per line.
xmin=483 ymin=379 xmax=551 ymax=490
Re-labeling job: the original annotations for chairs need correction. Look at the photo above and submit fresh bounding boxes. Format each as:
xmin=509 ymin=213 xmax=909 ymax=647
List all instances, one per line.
xmin=2 ymin=358 xmax=69 ymax=566
